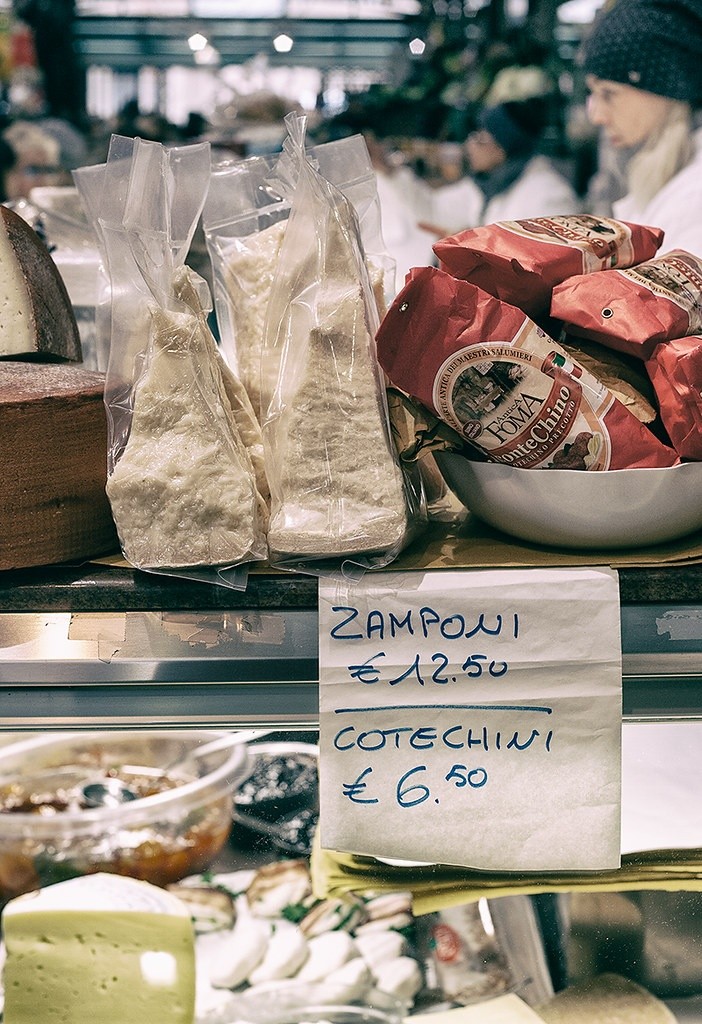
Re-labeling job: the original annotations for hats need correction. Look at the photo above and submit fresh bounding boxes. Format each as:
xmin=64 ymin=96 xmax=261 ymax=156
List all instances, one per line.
xmin=476 ymin=97 xmax=548 ymax=161
xmin=580 ymin=0 xmax=701 ymax=103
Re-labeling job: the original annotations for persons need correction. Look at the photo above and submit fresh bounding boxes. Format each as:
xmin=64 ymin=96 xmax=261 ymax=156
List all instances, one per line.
xmin=0 ymin=93 xmax=576 ymax=277
xmin=579 ymin=1 xmax=702 ymax=260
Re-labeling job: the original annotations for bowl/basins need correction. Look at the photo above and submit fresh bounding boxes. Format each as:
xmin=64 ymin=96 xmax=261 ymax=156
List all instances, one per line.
xmin=485 ymin=831 xmax=702 ymax=1006
xmin=427 ymin=435 xmax=701 ymax=556
xmin=0 ymin=731 xmax=252 ymax=908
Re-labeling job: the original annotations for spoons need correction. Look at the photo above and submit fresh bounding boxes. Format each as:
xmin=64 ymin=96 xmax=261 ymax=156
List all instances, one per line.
xmin=80 ymin=728 xmax=277 ymax=810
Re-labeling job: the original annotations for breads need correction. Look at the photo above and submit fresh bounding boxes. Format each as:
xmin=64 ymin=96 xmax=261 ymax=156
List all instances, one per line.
xmin=2 ymin=104 xmax=700 ymax=1024
